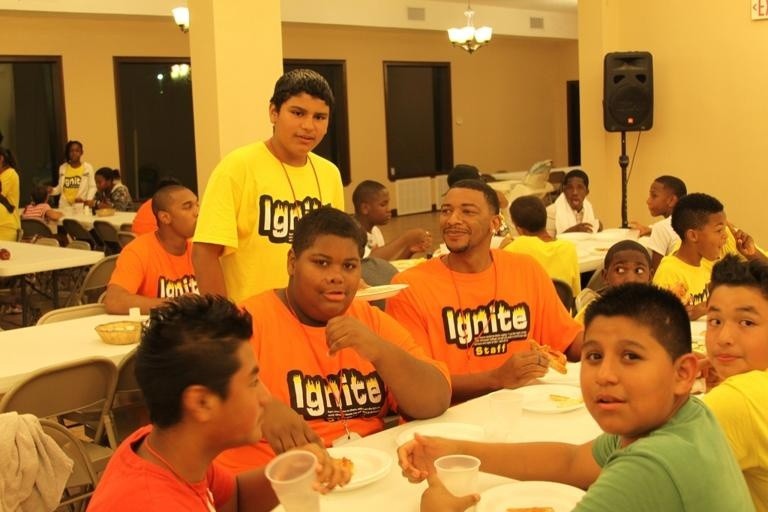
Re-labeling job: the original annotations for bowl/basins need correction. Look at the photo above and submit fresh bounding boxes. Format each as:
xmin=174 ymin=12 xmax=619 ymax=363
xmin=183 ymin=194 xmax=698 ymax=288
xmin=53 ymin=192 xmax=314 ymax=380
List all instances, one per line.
xmin=94 ymin=321 xmax=141 ymax=344
xmin=95 ymin=208 xmax=116 ymax=217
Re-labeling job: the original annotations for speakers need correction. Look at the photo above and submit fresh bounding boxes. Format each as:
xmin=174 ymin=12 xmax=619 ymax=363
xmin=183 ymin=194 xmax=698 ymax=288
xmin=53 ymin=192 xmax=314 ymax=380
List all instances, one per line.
xmin=601 ymin=51 xmax=656 ymax=134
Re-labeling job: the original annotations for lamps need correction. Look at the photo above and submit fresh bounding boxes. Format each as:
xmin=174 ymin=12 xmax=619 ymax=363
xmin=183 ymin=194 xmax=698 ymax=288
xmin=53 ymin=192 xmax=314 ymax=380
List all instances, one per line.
xmin=448 ymin=9 xmax=493 ymax=54
xmin=170 ymin=6 xmax=191 ymax=33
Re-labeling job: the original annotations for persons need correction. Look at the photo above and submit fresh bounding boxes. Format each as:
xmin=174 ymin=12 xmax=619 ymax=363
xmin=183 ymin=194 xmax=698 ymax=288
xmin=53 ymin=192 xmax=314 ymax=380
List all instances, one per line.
xmin=190 ymin=68 xmax=346 ymax=305
xmin=75 ymin=167 xmax=135 ymax=215
xmin=628 ymin=176 xmax=687 ymax=282
xmin=351 ymin=180 xmax=433 ymax=261
xmin=572 ymin=239 xmax=655 ymax=329
xmin=493 ymin=191 xmax=582 ymax=297
xmin=0 ymin=147 xmax=21 ymax=242
xmin=697 ymin=255 xmax=768 ymax=512
xmin=85 ymin=296 xmax=352 ymax=511
xmin=20 ymin=183 xmax=63 ymax=245
xmin=105 ymin=186 xmax=200 ymax=315
xmin=447 ymin=164 xmax=481 ymax=188
xmin=652 ymin=193 xmax=768 ymax=321
xmin=396 ymin=284 xmax=758 ymax=511
xmin=546 ymin=169 xmax=603 ymax=241
xmin=385 ymin=179 xmax=584 ymax=407
xmin=208 ymin=206 xmax=452 ymax=473
xmin=47 ymin=141 xmax=98 ymax=247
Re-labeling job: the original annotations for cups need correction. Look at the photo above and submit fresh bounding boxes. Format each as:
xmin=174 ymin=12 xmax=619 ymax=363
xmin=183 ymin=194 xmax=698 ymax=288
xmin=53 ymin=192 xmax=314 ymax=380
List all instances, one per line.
xmin=433 ymin=454 xmax=482 ymax=512
xmin=265 ymin=449 xmax=320 ymax=512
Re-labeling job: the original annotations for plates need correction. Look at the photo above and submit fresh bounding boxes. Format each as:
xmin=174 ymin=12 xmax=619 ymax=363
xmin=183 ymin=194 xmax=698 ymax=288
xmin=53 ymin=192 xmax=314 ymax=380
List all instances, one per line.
xmin=397 ymin=423 xmax=495 ymax=447
xmin=356 ymin=283 xmax=409 ymax=300
xmin=689 ymin=321 xmax=708 ymax=342
xmin=514 ymin=385 xmax=585 ymax=413
xmin=476 ymin=481 xmax=587 ymax=512
xmin=318 ymin=447 xmax=393 ymax=491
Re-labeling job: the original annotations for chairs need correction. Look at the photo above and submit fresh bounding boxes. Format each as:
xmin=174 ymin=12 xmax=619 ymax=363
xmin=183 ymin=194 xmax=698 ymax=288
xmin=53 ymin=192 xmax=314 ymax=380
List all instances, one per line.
xmin=119 ymin=222 xmax=134 ymax=230
xmin=92 ymin=219 xmax=117 ymax=247
xmin=63 ymin=219 xmax=88 ymax=239
xmin=35 ymin=303 xmax=106 ymax=328
xmin=114 ymin=231 xmax=136 ymax=247
xmin=68 ymin=254 xmax=118 ymax=303
xmin=0 ymin=415 xmax=98 ymax=512
xmin=20 ymin=217 xmax=53 ymax=236
xmin=0 ymin=355 xmax=119 ymax=446
xmin=117 ymin=341 xmax=145 ymax=440
xmin=69 ymin=241 xmax=90 ymax=248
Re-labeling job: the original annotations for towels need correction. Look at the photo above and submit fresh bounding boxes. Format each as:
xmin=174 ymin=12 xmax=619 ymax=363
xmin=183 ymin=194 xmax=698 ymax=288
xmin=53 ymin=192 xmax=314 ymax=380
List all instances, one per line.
xmin=556 ymin=192 xmax=600 ymax=235
xmin=0 ymin=411 xmax=74 ymax=512
